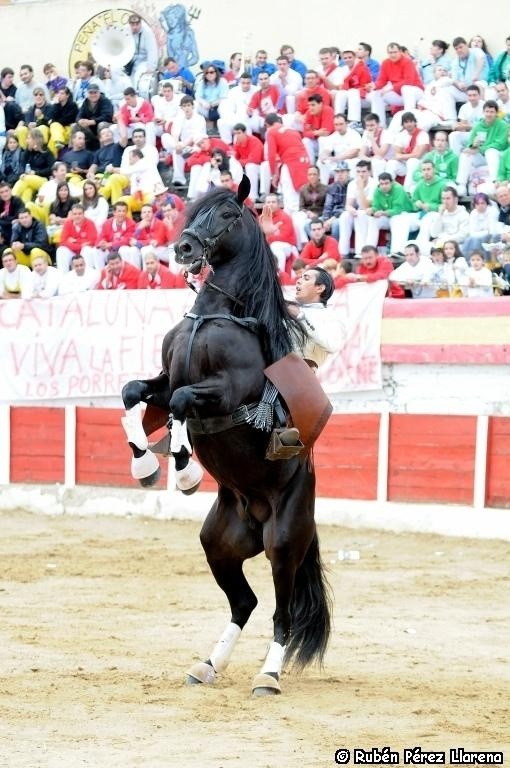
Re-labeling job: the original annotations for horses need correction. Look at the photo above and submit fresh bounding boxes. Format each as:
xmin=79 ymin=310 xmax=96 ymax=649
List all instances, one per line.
xmin=116 ymin=172 xmax=334 ymax=701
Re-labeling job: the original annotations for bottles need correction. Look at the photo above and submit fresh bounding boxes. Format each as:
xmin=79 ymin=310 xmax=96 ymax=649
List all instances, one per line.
xmin=25 ymin=163 xmax=31 ymax=174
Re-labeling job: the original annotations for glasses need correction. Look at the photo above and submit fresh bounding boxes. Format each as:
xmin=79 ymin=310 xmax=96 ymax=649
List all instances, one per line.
xmin=205 ymin=71 xmax=215 ymax=74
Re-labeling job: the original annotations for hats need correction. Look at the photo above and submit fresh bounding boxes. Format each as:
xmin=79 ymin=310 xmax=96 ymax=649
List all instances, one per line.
xmin=87 ymin=85 xmax=98 ymax=92
xmin=332 ymin=161 xmax=350 ymax=171
xmin=152 ymin=181 xmax=168 ymax=197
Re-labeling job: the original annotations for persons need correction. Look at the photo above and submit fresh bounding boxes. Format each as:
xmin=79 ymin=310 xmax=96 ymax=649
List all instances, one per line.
xmin=0 ymin=34 xmax=510 ymax=300
xmin=123 ymin=14 xmax=159 ymax=76
xmin=265 ymin=265 xmax=345 ymax=464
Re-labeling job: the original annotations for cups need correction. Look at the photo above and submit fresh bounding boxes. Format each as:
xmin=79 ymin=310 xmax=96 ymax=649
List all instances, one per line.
xmin=476 ymin=131 xmax=487 ymax=145
xmin=338 ymin=549 xmax=360 ymax=561
xmin=72 ymin=161 xmax=78 ymax=170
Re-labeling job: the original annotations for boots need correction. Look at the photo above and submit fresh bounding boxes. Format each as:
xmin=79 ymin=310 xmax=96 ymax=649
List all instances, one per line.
xmin=264 ymin=414 xmax=300 ymax=462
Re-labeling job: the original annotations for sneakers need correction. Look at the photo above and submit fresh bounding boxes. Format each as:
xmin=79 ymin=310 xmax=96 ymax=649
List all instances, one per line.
xmin=183 ymin=146 xmax=196 ymax=159
xmin=173 ymin=180 xmax=186 ymax=193
